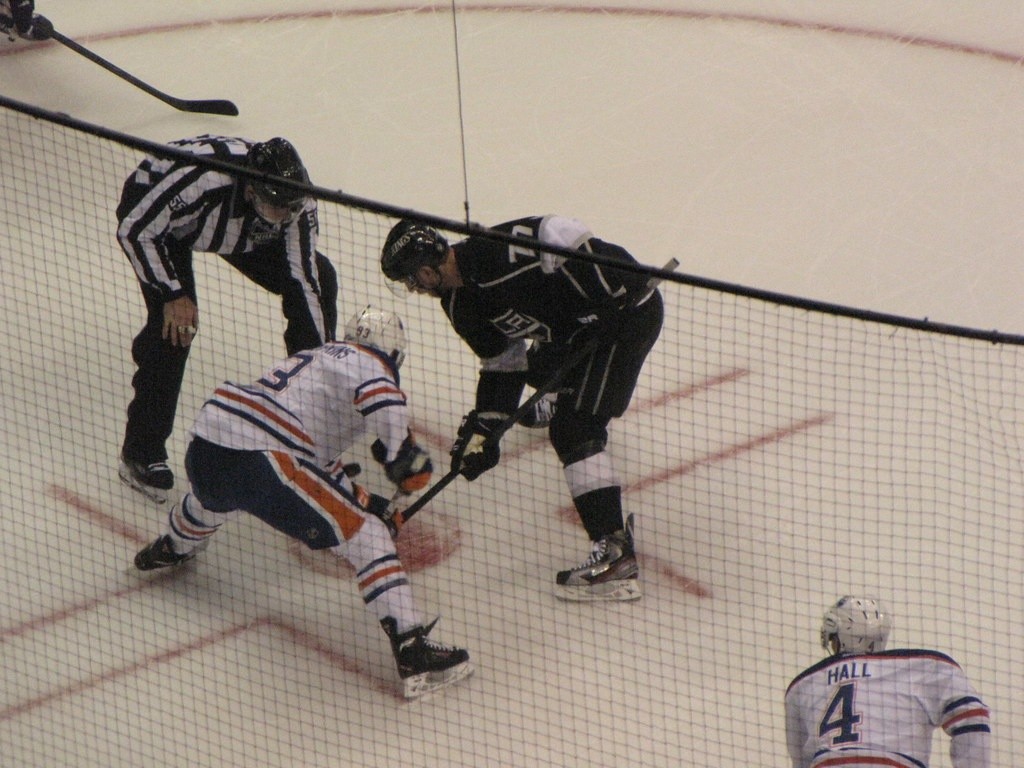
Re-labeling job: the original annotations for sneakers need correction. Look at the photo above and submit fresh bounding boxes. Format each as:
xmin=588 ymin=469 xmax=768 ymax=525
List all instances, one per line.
xmin=379 ymin=616 xmax=476 ymax=698
xmin=554 ymin=511 xmax=642 ymax=601
xmin=117 ymin=449 xmax=174 ymax=504
xmin=134 ymin=535 xmax=192 ymax=571
xmin=519 ymin=396 xmax=558 ymax=430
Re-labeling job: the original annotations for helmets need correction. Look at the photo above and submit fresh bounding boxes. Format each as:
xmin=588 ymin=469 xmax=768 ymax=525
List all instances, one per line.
xmin=819 ymin=594 xmax=892 ymax=655
xmin=245 ymin=137 xmax=311 ymax=219
xmin=344 ymin=305 xmax=407 ymax=367
xmin=380 ymin=217 xmax=449 ymax=300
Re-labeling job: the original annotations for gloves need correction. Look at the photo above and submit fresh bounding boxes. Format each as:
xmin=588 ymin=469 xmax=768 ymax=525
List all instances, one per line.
xmin=351 ymin=481 xmax=402 ymax=536
xmin=565 ymin=310 xmax=611 ymax=347
xmin=371 ymin=428 xmax=434 ymax=493
xmin=0 ymin=0 xmax=53 ymax=41
xmin=449 ymin=409 xmax=501 ymax=482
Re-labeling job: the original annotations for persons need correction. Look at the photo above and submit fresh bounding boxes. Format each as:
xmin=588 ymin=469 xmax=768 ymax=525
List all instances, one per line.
xmin=0 ymin=0 xmax=53 ymax=41
xmin=117 ymin=134 xmax=337 ymax=506
xmin=134 ymin=307 xmax=472 ymax=700
xmin=786 ymin=594 xmax=993 ymax=768
xmin=375 ymin=215 xmax=663 ymax=602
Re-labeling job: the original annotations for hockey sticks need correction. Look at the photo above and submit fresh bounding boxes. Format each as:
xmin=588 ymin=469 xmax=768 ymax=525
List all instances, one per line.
xmin=380 ymin=451 xmax=430 ymax=522
xmin=396 ymin=256 xmax=684 ymax=524
xmin=32 ymin=18 xmax=239 ymax=118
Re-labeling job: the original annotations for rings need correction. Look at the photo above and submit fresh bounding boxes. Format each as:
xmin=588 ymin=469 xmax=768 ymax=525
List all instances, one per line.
xmin=178 ymin=327 xmax=185 ymax=332
xmin=187 ymin=326 xmax=195 ymax=334
